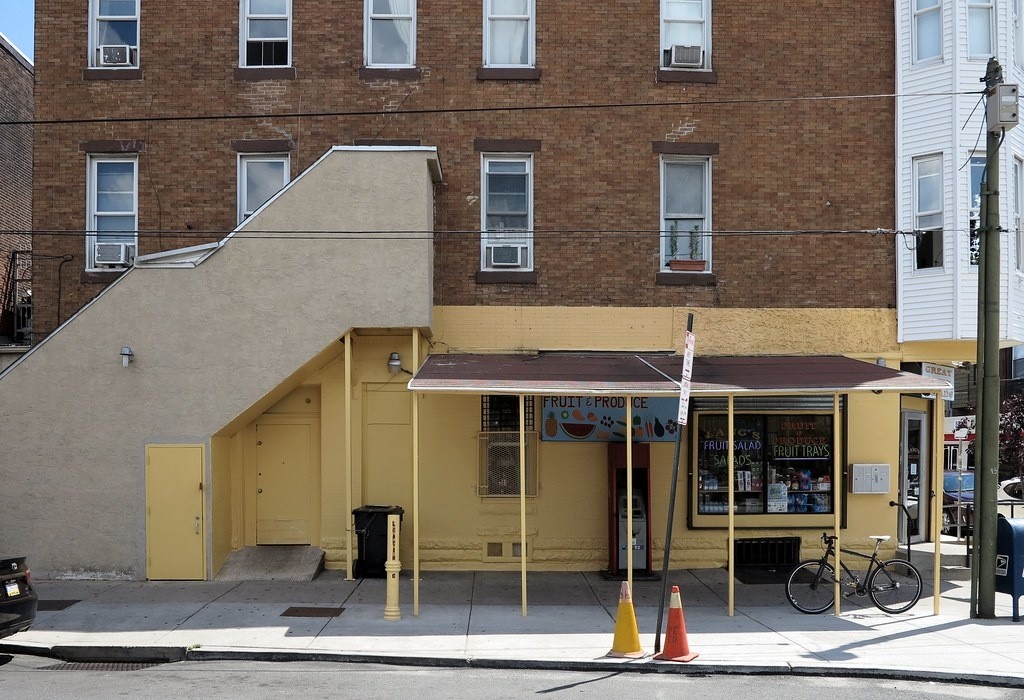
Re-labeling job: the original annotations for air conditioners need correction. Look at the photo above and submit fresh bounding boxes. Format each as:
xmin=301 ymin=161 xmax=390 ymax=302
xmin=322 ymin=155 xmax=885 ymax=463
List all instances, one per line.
xmin=668 ymin=44 xmax=703 ymax=68
xmin=491 ymin=245 xmax=521 ymax=267
xmin=95 ymin=243 xmax=129 ymax=264
xmin=99 ymin=45 xmax=135 ymax=66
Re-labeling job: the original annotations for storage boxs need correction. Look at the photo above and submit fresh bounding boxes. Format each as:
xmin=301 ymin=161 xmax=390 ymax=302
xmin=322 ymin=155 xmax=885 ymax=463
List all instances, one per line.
xmin=737 ymin=471 xmax=751 ymax=491
xmin=817 ymin=483 xmax=831 ymax=490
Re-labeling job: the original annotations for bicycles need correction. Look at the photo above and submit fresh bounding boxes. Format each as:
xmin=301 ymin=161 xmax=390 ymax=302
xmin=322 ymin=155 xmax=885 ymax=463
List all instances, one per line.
xmin=781 ymin=530 xmax=924 ymax=614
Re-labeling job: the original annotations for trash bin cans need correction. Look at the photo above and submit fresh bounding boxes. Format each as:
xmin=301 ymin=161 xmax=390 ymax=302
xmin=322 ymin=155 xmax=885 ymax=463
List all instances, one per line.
xmin=995 ymin=513 xmax=1024 ymax=622
xmin=352 ymin=504 xmax=405 ymax=578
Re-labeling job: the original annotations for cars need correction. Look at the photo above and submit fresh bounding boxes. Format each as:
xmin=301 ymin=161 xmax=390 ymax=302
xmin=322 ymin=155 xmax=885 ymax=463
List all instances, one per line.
xmin=1 ymin=552 xmax=36 ymax=641
xmin=908 ymin=469 xmax=998 ymax=537
xmin=995 ymin=475 xmax=1024 ymax=522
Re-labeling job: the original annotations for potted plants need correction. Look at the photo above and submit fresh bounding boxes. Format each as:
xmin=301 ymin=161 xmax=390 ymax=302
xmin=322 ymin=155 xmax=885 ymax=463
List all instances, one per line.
xmin=668 ymin=220 xmax=707 ymax=272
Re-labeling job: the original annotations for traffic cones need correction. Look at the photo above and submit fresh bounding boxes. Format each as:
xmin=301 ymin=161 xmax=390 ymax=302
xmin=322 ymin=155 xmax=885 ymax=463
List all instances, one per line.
xmin=654 ymin=585 xmax=700 ymax=662
xmin=607 ymin=580 xmax=648 ymax=659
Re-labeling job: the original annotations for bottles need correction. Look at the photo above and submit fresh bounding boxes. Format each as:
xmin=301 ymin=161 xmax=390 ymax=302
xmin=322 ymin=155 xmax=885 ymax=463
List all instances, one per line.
xmin=778 ymin=475 xmax=784 ymax=483
xmin=785 ymin=474 xmax=791 ymax=491
xmin=699 ymin=476 xmax=703 ymax=491
xmin=792 ymin=475 xmax=799 ymax=490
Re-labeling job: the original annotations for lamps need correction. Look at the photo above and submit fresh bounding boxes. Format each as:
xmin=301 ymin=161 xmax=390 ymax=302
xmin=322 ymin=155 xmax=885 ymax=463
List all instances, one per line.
xmin=119 ymin=346 xmax=134 ymax=368
xmin=387 ymin=352 xmax=402 ymax=374
